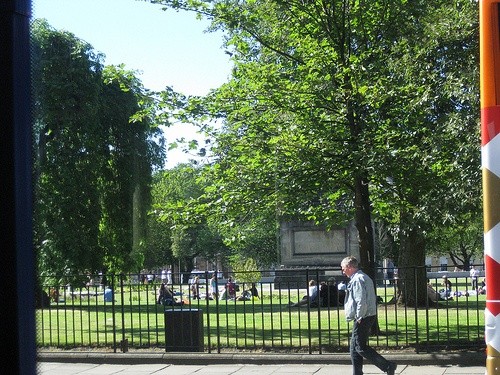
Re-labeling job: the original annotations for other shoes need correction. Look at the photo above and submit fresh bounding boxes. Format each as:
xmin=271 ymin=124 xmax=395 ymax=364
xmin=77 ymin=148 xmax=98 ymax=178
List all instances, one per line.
xmin=387 ymin=362 xmax=397 ymax=375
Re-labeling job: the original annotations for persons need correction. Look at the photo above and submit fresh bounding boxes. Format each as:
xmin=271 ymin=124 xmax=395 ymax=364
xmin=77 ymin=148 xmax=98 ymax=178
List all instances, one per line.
xmin=99 ymin=273 xmax=109 ymax=292
xmin=286 ymin=277 xmax=347 ymax=307
xmin=190 ymin=266 xmax=261 ymax=302
xmin=386 ymin=259 xmax=395 ymax=285
xmin=48 ymin=286 xmax=60 ymax=303
xmin=453 ymin=266 xmax=459 ymax=273
xmin=426 ymin=275 xmax=486 ymax=301
xmin=139 ymin=266 xmax=176 ymax=285
xmin=103 ymin=286 xmax=114 ymax=302
xmin=468 ymin=266 xmax=480 ymax=290
xmin=341 ymin=257 xmax=397 ymax=375
xmin=157 ymin=283 xmax=184 ymax=306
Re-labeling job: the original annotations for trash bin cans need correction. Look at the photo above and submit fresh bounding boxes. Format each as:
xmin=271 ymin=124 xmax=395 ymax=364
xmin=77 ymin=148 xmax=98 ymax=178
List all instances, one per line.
xmin=164 ymin=309 xmax=204 ymax=352
